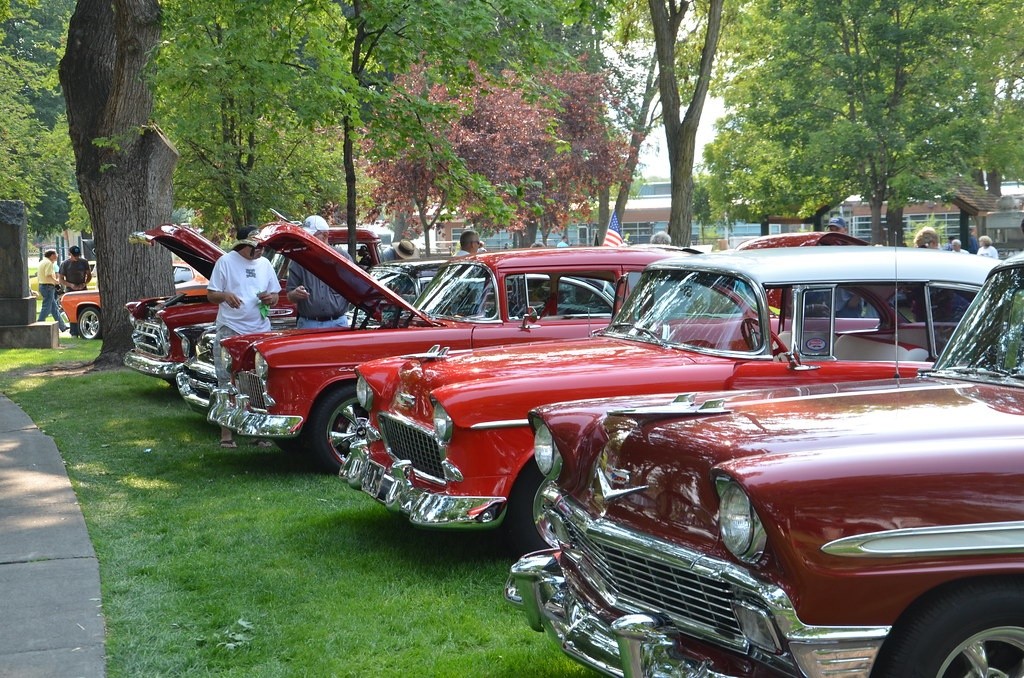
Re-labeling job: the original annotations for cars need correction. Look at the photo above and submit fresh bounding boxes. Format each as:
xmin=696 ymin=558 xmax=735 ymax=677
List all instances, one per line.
xmin=61 ymin=262 xmax=209 ymax=340
xmin=336 ymin=245 xmax=1005 ymax=565
xmin=502 ymin=249 xmax=1024 ymax=677
xmin=121 ymin=209 xmax=882 ymax=476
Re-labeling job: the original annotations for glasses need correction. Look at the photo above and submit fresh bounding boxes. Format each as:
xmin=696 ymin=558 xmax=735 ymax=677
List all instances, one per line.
xmin=472 ymin=241 xmax=480 ymax=245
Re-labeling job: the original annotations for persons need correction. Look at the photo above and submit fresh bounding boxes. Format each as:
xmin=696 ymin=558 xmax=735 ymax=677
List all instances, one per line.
xmin=977 ymin=236 xmax=999 ymax=258
xmin=951 ymin=239 xmax=969 ymax=254
xmin=59 ymin=246 xmax=92 ymax=337
xmin=38 ymin=250 xmax=70 ymax=332
xmin=823 ymin=217 xmax=864 ymax=317
xmin=557 ymin=236 xmax=568 ymax=247
xmin=385 ymin=240 xmax=420 ymax=259
xmin=477 ymin=241 xmax=486 ymax=251
xmin=910 ymin=226 xmax=953 ymax=321
xmin=455 ymin=230 xmax=480 ymax=256
xmin=650 ymin=232 xmax=671 ymax=244
xmin=969 ymin=229 xmax=980 ymax=253
xmin=206 ymin=224 xmax=281 ymax=448
xmin=54 ymin=263 xmax=61 ymax=290
xmin=505 ymin=243 xmax=509 ymax=249
xmin=286 ymin=216 xmax=352 ymax=328
xmin=945 ymin=237 xmax=955 ymax=251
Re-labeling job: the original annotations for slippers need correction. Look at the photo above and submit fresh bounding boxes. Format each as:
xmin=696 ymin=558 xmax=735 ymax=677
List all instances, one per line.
xmin=249 ymin=438 xmax=272 ymax=448
xmin=219 ymin=440 xmax=238 ymax=449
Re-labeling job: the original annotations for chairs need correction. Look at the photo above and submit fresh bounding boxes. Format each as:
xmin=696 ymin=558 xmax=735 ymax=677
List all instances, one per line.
xmin=765 ymin=330 xmax=839 ymax=358
xmin=832 ymin=334 xmax=928 ymax=362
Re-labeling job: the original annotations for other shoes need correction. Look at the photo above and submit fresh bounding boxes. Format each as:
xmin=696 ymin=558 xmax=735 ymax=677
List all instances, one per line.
xmin=62 ymin=327 xmax=70 ymax=332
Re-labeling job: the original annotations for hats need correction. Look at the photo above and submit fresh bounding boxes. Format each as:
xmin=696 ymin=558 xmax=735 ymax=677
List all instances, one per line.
xmin=233 ymin=225 xmax=260 ymax=252
xmin=70 ymin=246 xmax=81 ymax=256
xmin=827 ymin=217 xmax=846 ymax=229
xmin=303 ymin=215 xmax=329 ymax=235
xmin=392 ymin=239 xmax=420 ymax=259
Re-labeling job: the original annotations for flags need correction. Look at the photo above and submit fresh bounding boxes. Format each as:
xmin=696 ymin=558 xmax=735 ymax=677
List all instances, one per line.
xmin=603 ymin=211 xmax=622 ymax=246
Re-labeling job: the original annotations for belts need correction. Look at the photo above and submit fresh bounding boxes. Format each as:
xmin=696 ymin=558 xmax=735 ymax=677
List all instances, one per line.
xmin=300 ymin=313 xmax=344 ymax=321
xmin=39 ymin=284 xmax=53 ymax=285
